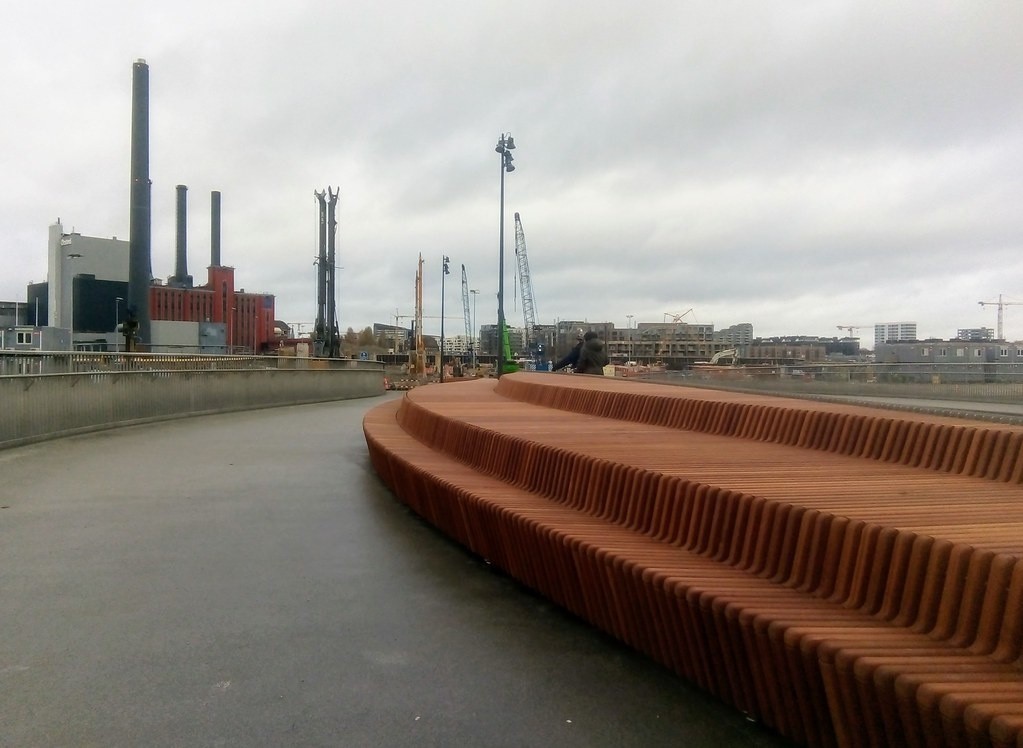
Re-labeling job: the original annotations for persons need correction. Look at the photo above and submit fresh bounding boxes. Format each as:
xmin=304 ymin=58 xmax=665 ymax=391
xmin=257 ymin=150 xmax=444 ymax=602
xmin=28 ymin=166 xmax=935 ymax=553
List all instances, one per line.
xmin=552 ymin=334 xmax=585 ymax=372
xmin=576 ymin=332 xmax=609 ymax=375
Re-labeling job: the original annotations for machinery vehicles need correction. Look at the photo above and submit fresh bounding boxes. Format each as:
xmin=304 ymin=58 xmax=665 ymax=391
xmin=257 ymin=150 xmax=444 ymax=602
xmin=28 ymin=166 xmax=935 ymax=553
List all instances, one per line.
xmin=461 ymin=264 xmax=481 ymax=369
xmin=514 ymin=212 xmax=554 ymax=372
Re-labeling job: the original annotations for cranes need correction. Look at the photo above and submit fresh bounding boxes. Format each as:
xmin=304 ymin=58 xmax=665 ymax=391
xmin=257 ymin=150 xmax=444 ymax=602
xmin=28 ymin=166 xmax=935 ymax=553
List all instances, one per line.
xmin=663 ymin=308 xmax=696 ymax=323
xmin=391 ymin=308 xmax=464 ymax=353
xmin=836 ymin=325 xmax=876 ymax=337
xmin=977 ymin=294 xmax=1023 ymax=340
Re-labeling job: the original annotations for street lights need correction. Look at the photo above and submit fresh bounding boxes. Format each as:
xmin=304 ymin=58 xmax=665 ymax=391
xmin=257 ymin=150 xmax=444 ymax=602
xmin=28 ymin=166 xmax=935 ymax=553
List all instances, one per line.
xmin=626 ymin=314 xmax=634 ymax=363
xmin=495 ymin=130 xmax=517 ymax=379
xmin=254 ymin=316 xmax=258 ymax=355
xmin=231 ymin=307 xmax=236 ymax=355
xmin=470 ymin=289 xmax=479 ymax=356
xmin=438 ymin=255 xmax=451 ymax=383
xmin=116 ymin=297 xmax=123 ymax=352
